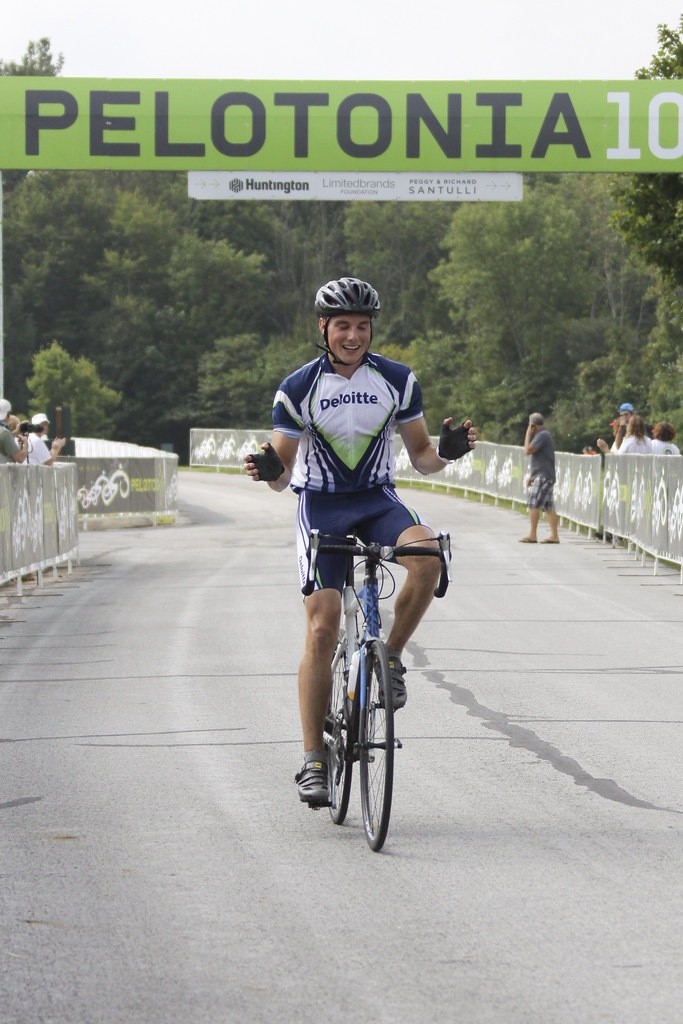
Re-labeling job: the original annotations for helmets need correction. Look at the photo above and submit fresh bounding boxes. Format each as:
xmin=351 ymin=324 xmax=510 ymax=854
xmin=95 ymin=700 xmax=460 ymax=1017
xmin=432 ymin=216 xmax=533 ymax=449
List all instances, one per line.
xmin=315 ymin=276 xmax=381 ymax=319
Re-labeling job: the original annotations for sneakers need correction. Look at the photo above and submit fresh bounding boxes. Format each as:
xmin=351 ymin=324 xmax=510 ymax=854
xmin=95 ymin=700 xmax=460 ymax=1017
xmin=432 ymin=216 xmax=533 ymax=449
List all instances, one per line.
xmin=294 ymin=760 xmax=330 ymax=803
xmin=374 ymin=655 xmax=407 ymax=710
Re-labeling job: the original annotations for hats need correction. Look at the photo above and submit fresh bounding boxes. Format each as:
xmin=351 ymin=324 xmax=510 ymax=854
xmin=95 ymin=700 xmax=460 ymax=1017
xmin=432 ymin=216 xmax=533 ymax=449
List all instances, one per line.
xmin=32 ymin=413 xmax=50 ymax=425
xmin=0 ymin=399 xmax=11 ymax=420
xmin=617 ymin=403 xmax=634 ymax=413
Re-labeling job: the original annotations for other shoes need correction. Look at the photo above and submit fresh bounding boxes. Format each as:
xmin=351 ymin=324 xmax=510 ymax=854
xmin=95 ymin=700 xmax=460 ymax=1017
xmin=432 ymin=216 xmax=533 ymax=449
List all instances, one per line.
xmin=519 ymin=537 xmax=538 ymax=543
xmin=540 ymin=538 xmax=559 ymax=543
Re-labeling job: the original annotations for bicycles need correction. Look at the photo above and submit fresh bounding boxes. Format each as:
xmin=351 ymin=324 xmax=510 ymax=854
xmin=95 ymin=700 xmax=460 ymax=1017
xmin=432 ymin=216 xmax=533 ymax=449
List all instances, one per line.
xmin=299 ymin=525 xmax=453 ymax=849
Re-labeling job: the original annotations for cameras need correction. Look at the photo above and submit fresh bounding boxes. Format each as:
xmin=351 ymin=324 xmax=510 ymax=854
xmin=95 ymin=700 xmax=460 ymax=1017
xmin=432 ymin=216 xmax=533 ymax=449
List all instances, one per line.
xmin=19 ymin=421 xmax=43 ymax=437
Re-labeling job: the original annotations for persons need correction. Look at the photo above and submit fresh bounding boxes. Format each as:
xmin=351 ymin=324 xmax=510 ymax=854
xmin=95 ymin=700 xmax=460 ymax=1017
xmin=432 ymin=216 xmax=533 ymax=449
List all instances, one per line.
xmin=650 ymin=423 xmax=680 ymax=455
xmin=5 ymin=415 xmax=20 ymax=447
xmin=617 ymin=415 xmax=652 ymax=452
xmin=27 ymin=413 xmax=67 ymax=466
xmin=242 ymin=278 xmax=478 ymax=800
xmin=596 ymin=418 xmax=619 ymax=453
xmin=517 ymin=412 xmax=560 ymax=544
xmin=615 ymin=403 xmax=634 ymax=449
xmin=582 ymin=445 xmax=596 ymax=455
xmin=0 ymin=398 xmax=29 ymax=463
